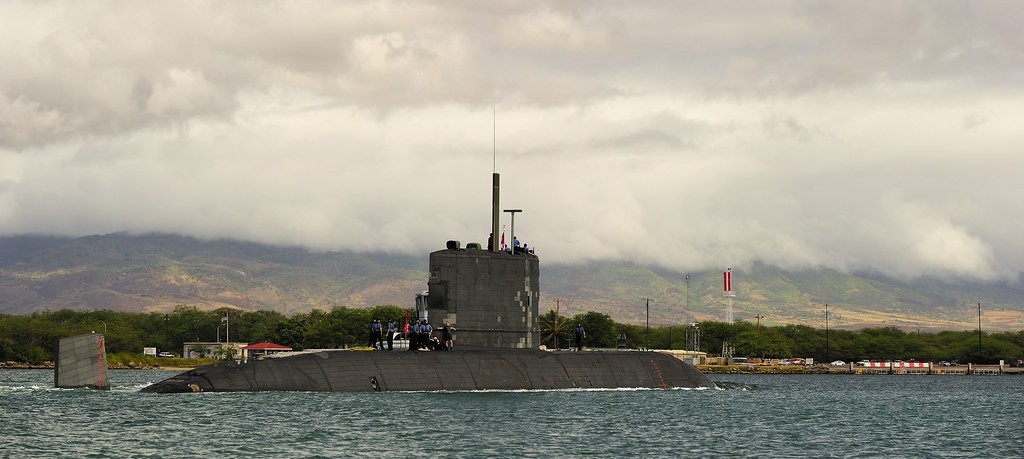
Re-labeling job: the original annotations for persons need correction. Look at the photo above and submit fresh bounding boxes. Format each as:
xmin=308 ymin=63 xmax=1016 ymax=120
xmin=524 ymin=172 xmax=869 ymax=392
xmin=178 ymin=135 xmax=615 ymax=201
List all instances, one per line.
xmin=513 ymin=235 xmax=530 ymax=252
xmin=575 ymin=321 xmax=586 ymax=350
xmin=505 ymin=244 xmax=510 ymax=250
xmin=368 ymin=318 xmax=394 ymax=350
xmin=409 ymin=319 xmax=457 ymax=351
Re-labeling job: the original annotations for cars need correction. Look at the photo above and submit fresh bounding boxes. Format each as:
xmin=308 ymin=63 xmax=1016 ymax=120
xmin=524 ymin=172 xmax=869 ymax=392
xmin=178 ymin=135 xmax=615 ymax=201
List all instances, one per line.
xmin=159 ymin=352 xmax=174 ymax=358
xmin=371 ymin=331 xmax=410 ymax=350
